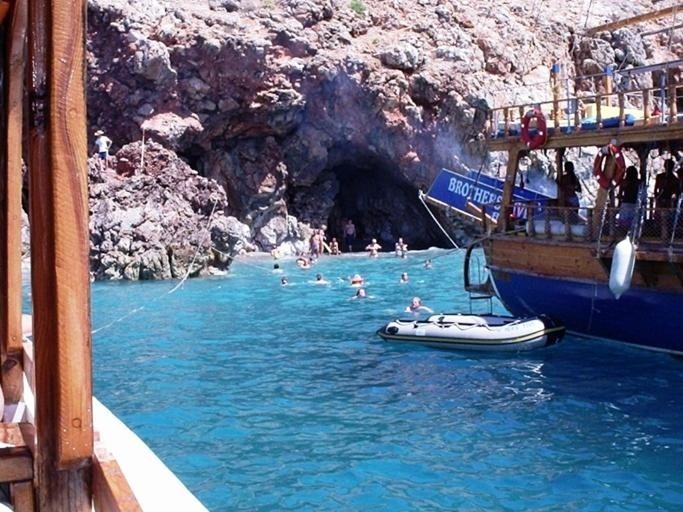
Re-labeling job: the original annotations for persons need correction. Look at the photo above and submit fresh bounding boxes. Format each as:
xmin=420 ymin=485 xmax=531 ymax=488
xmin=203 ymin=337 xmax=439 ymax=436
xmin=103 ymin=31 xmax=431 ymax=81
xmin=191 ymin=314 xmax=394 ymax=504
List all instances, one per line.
xmin=272 ymin=219 xmax=431 ymax=288
xmin=618 ymin=166 xmax=641 ymax=232
xmin=406 ymin=296 xmax=432 ymax=313
xmin=352 ymin=288 xmax=370 ymax=298
xmin=91 ymin=130 xmax=112 ymax=169
xmin=654 ymin=160 xmax=680 ymax=239
xmin=561 ymin=162 xmax=581 ymax=222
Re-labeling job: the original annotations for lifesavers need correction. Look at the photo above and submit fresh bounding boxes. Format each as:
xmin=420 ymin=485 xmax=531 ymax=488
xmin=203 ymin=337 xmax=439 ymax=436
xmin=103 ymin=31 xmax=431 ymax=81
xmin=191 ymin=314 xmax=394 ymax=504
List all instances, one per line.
xmin=520 ymin=109 xmax=548 ymax=149
xmin=593 ymin=144 xmax=625 ymax=189
